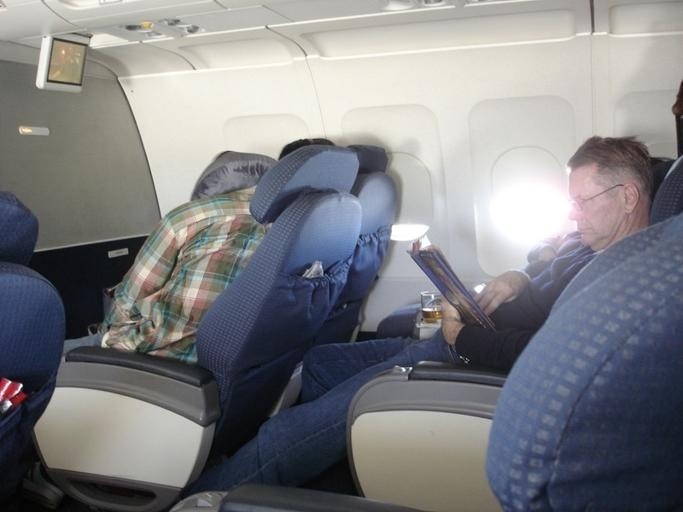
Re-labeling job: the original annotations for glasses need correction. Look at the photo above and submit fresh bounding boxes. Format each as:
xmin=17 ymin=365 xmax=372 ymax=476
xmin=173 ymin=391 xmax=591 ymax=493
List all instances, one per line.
xmin=569 ymin=183 xmax=625 ymax=211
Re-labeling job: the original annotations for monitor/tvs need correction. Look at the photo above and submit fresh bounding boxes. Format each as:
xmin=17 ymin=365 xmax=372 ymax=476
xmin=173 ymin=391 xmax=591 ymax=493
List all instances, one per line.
xmin=35 ymin=34 xmax=90 ymax=94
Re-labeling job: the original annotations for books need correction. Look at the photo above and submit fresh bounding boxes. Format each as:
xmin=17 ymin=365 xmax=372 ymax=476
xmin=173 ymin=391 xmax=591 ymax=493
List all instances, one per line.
xmin=407 ymin=234 xmax=499 ymax=334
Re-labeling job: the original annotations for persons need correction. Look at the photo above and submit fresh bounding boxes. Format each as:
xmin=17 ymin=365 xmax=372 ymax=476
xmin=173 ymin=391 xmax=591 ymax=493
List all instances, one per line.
xmin=178 ymin=135 xmax=654 ymax=502
xmin=90 ymin=135 xmax=338 ymax=366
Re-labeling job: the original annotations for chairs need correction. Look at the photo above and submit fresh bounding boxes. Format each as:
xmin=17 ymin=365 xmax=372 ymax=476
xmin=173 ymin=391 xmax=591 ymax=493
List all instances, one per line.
xmin=223 ymin=155 xmax=682 ymax=511
xmin=2 ymin=185 xmax=69 ymax=509
xmin=36 ymin=144 xmax=398 ymax=511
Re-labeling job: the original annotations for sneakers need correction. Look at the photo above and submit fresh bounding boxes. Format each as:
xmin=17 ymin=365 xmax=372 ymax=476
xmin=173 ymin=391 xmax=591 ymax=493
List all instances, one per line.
xmin=23 ymin=462 xmax=64 ymax=511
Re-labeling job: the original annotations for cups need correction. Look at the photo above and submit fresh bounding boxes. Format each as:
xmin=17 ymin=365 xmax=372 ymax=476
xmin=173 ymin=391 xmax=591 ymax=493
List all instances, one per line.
xmin=420 ymin=290 xmax=444 ymax=324
